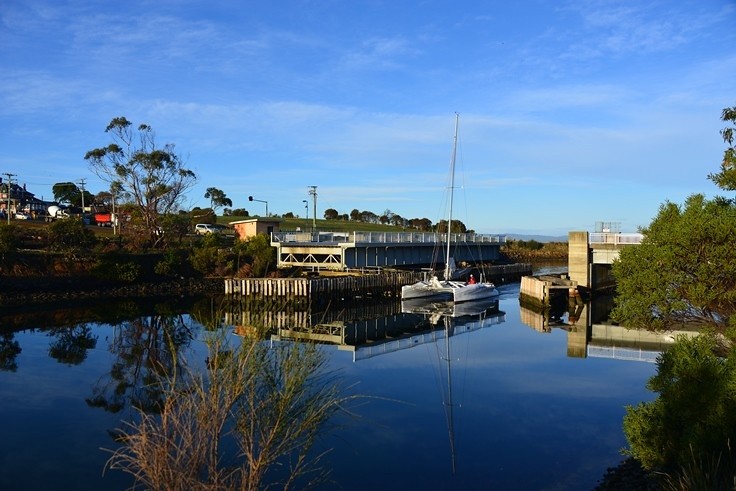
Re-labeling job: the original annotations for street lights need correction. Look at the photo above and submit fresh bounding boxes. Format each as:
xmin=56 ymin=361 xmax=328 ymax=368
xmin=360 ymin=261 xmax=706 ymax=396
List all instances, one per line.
xmin=302 ymin=199 xmax=309 ymax=233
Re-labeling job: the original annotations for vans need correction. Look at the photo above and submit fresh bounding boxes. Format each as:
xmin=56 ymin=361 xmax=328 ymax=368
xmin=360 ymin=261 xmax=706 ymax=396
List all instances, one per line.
xmin=194 ymin=224 xmax=220 ymax=235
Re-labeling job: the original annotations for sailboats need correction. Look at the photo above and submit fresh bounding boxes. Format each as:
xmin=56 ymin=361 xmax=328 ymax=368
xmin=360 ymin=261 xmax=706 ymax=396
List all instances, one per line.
xmin=404 ymin=112 xmax=500 ymax=323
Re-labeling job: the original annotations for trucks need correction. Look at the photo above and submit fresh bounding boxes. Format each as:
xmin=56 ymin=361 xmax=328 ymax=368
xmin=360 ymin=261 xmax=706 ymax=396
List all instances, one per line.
xmin=95 ymin=213 xmax=123 ymax=227
xmin=43 ymin=206 xmax=72 ymax=222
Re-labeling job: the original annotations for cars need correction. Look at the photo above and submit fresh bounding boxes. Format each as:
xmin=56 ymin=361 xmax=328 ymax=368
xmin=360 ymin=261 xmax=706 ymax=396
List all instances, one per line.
xmin=15 ymin=212 xmax=31 ymax=219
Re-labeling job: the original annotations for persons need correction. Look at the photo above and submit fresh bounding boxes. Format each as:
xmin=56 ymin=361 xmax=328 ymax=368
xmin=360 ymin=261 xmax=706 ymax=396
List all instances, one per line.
xmin=467 ymin=274 xmax=478 ymax=285
